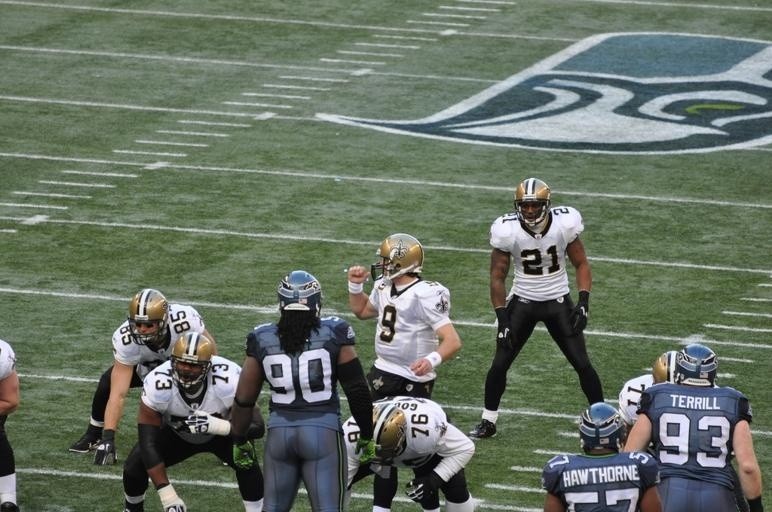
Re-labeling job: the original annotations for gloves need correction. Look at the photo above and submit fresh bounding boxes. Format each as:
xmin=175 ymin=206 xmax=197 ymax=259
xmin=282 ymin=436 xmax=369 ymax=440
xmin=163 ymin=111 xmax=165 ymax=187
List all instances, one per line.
xmin=495 ymin=307 xmax=512 ymax=349
xmin=93 ymin=429 xmax=117 ymax=465
xmin=183 ymin=410 xmax=230 ymax=436
xmin=569 ymin=291 xmax=589 ymax=335
xmin=157 ymin=483 xmax=187 ymax=512
xmin=355 ymin=438 xmax=375 ymax=463
xmin=405 ymin=471 xmax=446 ymax=502
xmin=232 ymin=436 xmax=256 ymax=470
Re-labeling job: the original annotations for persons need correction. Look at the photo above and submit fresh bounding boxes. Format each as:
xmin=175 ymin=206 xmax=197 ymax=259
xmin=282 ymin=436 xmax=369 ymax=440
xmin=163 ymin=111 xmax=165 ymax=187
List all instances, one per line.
xmin=0 ymin=338 xmax=20 ymax=512
xmin=68 ymin=289 xmax=228 ymax=467
xmin=122 ymin=332 xmax=264 ymax=512
xmin=228 ymin=271 xmax=377 ymax=512
xmin=347 ymin=233 xmax=462 ymax=512
xmin=467 ymin=178 xmax=605 ymax=440
xmin=342 ymin=396 xmax=476 ymax=512
xmin=541 ymin=344 xmax=763 ymax=512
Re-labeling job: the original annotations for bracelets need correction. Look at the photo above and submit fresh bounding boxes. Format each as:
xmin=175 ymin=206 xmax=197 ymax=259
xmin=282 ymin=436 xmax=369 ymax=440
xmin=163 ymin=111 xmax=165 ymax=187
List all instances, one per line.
xmin=347 ymin=280 xmax=363 ymax=294
xmin=426 ymin=351 xmax=442 ymax=368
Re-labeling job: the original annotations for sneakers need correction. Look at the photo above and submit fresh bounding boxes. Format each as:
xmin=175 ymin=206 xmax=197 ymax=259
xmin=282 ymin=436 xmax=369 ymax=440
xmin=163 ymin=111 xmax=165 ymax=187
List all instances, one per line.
xmin=1 ymin=502 xmax=20 ymax=512
xmin=468 ymin=418 xmax=497 ymax=441
xmin=69 ymin=432 xmax=102 ymax=453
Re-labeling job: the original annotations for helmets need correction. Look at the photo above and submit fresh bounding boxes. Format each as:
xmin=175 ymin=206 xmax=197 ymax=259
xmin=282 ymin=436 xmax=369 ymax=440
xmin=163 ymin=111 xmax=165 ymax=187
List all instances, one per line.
xmin=515 ymin=178 xmax=551 ymax=226
xmin=128 ymin=289 xmax=169 ymax=345
xmin=278 ymin=271 xmax=322 ymax=319
xmin=675 ymin=344 xmax=717 ymax=387
xmin=371 ymin=233 xmax=423 ymax=281
xmin=653 ymin=350 xmax=679 ymax=384
xmin=579 ymin=402 xmax=627 ymax=452
xmin=171 ymin=332 xmax=211 ymax=385
xmin=372 ymin=404 xmax=407 ymax=457
